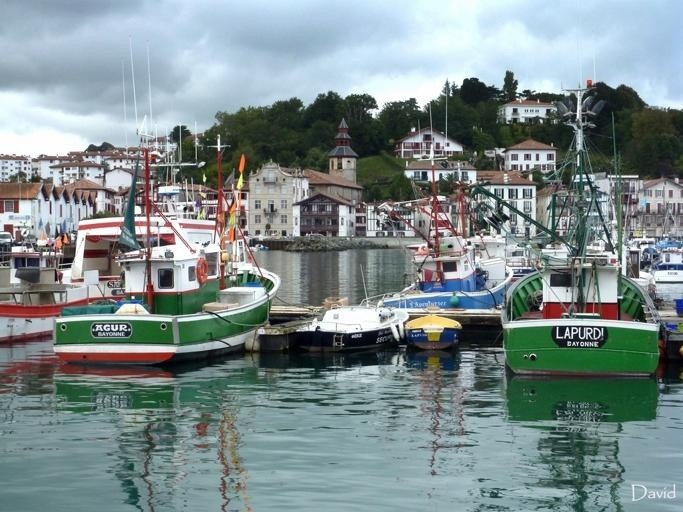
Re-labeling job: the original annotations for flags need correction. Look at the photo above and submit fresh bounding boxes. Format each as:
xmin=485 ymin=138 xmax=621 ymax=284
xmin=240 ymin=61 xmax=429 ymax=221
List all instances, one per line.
xmin=114 ymin=154 xmax=138 ymax=249
xmin=225 ymin=153 xmax=245 ymax=243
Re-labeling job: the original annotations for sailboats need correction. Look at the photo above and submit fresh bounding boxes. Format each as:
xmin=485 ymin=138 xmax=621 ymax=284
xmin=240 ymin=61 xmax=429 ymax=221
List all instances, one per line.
xmin=0 ymin=83 xmax=683 ymax=376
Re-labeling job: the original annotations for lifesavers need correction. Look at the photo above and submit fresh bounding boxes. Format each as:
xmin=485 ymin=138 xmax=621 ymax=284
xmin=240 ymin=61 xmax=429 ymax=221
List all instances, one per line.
xmin=197 ymin=258 xmax=208 ymax=284
